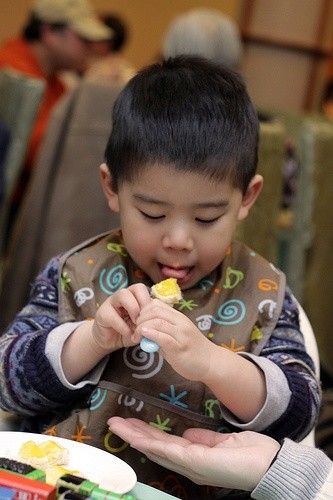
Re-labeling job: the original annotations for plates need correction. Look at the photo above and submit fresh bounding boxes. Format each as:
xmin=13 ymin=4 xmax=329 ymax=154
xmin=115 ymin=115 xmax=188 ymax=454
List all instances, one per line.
xmin=1 ymin=431 xmax=137 ymax=494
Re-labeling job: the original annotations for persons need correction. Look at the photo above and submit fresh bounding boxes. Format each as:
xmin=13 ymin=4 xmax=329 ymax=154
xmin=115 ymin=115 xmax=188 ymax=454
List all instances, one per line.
xmin=1 ymin=52 xmax=321 ymax=500
xmin=0 ymin=1 xmax=114 ymax=204
xmin=320 ymin=77 xmax=333 ymax=123
xmin=154 ymin=12 xmax=299 ymax=278
xmin=60 ymin=12 xmax=136 ymax=84
xmin=102 ymin=416 xmax=333 ymax=500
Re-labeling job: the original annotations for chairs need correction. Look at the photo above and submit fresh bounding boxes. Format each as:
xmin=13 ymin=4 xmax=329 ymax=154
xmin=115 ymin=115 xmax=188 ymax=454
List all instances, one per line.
xmin=0 ymin=67 xmax=332 ymax=374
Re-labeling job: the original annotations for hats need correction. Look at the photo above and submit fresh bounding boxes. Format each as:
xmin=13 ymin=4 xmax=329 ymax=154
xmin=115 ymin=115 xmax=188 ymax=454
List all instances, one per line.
xmin=33 ymin=1 xmax=112 ymax=42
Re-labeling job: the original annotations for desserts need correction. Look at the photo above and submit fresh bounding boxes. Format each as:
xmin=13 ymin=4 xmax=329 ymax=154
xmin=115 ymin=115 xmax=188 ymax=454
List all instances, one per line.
xmin=18 ymin=441 xmax=81 ymax=486
xmin=148 ymin=277 xmax=182 ymax=307
